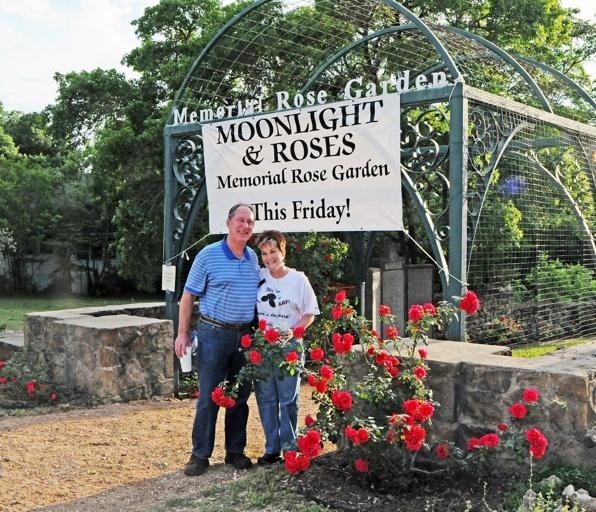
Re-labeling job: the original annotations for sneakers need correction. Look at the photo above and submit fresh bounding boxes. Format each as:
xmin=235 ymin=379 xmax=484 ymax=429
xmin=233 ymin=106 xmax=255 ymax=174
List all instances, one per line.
xmin=225 ymin=452 xmax=252 ymax=469
xmin=257 ymin=452 xmax=282 ymax=465
xmin=184 ymin=455 xmax=209 ymax=475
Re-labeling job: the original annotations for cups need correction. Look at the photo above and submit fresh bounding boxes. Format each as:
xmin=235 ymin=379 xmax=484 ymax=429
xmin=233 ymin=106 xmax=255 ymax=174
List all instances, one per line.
xmin=179 ymin=346 xmax=193 ymax=373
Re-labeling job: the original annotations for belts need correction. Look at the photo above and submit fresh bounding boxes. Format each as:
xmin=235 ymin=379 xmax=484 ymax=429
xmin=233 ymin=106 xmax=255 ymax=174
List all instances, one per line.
xmin=199 ymin=315 xmax=251 ymax=331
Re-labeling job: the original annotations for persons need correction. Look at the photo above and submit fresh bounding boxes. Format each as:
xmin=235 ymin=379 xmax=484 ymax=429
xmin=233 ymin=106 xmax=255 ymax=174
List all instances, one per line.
xmin=248 ymin=230 xmax=320 ymax=465
xmin=174 ymin=203 xmax=261 ymax=476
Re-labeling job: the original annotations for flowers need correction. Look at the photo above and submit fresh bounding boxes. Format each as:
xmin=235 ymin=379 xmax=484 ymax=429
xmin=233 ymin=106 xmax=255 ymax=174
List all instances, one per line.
xmin=197 ymin=284 xmax=547 ymax=477
